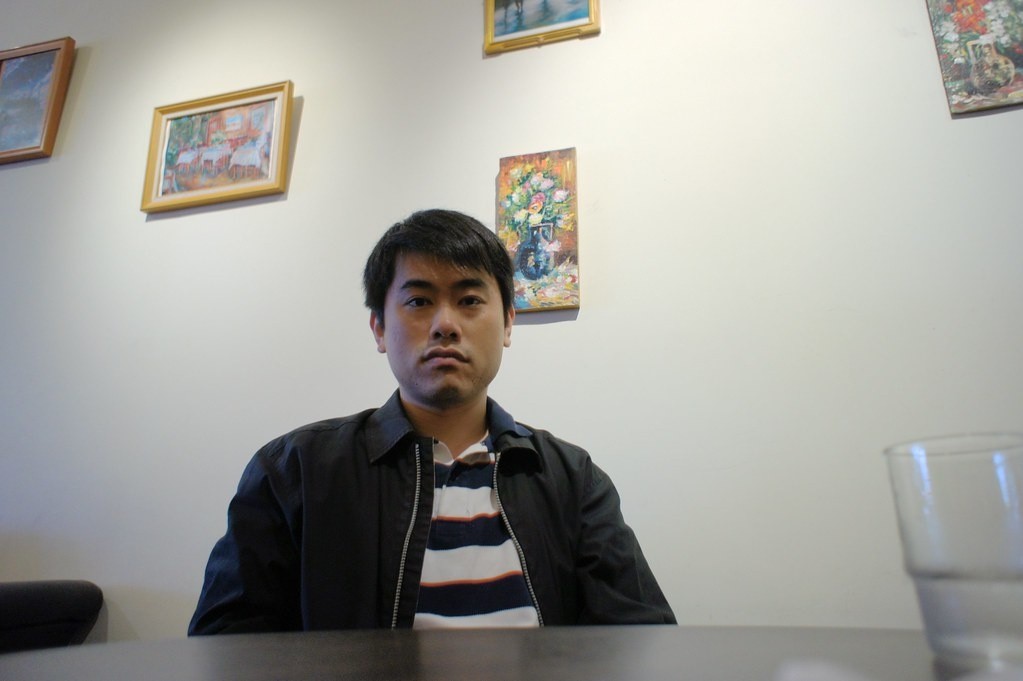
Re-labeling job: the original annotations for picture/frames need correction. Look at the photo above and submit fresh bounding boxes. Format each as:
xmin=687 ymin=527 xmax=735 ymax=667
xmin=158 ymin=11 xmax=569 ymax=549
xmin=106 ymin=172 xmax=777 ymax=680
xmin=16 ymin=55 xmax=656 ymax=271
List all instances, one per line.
xmin=0 ymin=36 xmax=76 ymax=166
xmin=483 ymin=0 xmax=601 ymax=55
xmin=140 ymin=80 xmax=292 ymax=213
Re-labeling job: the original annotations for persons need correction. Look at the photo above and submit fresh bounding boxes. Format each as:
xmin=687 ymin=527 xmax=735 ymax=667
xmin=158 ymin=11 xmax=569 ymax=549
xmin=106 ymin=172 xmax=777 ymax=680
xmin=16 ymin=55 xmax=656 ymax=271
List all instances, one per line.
xmin=183 ymin=209 xmax=678 ymax=638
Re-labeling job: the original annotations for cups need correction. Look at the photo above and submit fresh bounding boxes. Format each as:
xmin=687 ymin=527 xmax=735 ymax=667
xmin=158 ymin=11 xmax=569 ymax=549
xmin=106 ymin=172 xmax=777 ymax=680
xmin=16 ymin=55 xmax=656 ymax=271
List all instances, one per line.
xmin=884 ymin=430 xmax=1023 ymax=681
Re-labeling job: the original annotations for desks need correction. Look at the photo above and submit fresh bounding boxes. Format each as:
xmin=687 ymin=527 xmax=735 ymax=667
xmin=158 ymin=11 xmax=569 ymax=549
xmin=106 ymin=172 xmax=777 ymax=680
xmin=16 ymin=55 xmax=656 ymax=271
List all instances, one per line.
xmin=156 ymin=628 xmax=931 ymax=681
